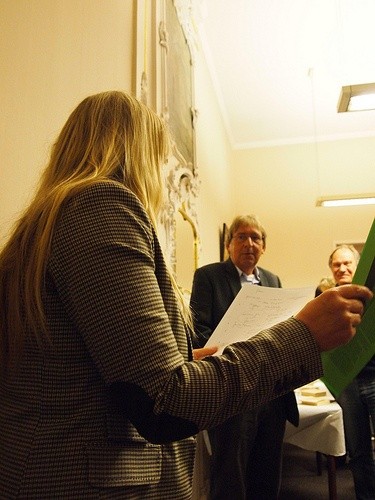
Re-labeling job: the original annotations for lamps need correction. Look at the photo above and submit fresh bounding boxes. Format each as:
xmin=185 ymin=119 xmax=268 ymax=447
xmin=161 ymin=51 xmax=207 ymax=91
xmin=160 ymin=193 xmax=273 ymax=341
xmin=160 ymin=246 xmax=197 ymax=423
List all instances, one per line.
xmin=337 ymin=83 xmax=375 ymax=112
xmin=316 ymin=193 xmax=375 ymax=207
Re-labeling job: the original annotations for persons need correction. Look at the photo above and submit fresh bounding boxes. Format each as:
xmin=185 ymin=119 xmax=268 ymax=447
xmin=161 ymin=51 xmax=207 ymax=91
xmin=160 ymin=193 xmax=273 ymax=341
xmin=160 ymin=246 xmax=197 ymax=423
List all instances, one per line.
xmin=190 ymin=215 xmax=299 ymax=500
xmin=1 ymin=90 xmax=372 ymax=500
xmin=314 ymin=245 xmax=361 ymax=298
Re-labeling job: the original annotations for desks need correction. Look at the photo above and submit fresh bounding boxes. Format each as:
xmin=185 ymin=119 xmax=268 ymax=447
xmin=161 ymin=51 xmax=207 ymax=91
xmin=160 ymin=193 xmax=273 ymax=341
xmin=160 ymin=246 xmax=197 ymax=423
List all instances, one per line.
xmin=285 ymin=379 xmax=346 ymax=500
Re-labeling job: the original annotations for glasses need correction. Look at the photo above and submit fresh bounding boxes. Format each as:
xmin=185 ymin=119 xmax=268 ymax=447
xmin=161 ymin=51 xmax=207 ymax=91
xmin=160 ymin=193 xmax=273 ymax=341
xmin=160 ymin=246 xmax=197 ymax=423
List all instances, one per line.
xmin=232 ymin=234 xmax=264 ymax=244
